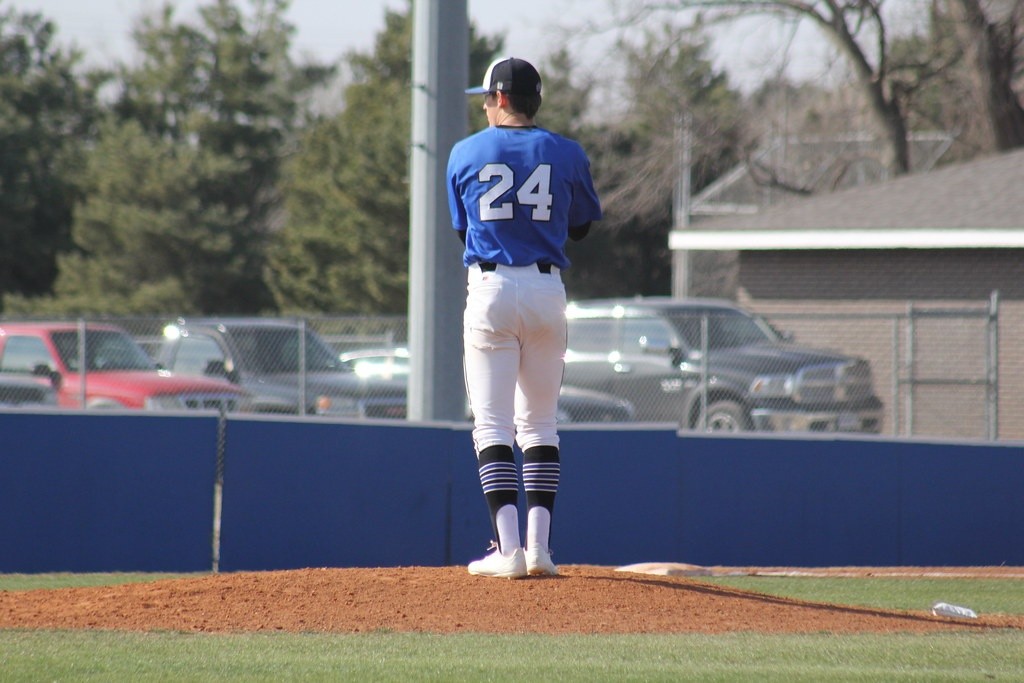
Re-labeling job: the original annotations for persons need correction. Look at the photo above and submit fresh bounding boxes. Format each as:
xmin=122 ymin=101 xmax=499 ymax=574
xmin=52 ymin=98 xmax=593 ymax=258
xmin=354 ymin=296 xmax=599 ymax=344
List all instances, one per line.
xmin=446 ymin=58 xmax=604 ymax=578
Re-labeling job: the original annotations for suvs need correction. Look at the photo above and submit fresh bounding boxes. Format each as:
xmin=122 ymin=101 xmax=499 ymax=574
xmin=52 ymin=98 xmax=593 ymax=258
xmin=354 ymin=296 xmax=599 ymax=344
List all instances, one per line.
xmin=0 ymin=319 xmax=243 ymax=420
xmin=559 ymin=297 xmax=885 ymax=433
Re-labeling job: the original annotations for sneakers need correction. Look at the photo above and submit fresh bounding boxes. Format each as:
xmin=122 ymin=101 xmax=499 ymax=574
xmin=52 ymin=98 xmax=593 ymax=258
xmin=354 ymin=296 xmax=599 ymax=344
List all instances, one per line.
xmin=522 ymin=543 xmax=559 ymax=576
xmin=467 ymin=544 xmax=527 ymax=580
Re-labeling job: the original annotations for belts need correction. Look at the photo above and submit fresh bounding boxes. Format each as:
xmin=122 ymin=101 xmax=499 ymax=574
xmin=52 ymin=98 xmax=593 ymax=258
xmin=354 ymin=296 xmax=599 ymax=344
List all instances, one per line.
xmin=478 ymin=262 xmax=552 ymax=273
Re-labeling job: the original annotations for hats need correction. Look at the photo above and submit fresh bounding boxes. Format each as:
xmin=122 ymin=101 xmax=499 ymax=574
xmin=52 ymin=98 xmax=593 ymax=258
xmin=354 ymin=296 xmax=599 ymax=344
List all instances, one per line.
xmin=464 ymin=57 xmax=542 ymax=95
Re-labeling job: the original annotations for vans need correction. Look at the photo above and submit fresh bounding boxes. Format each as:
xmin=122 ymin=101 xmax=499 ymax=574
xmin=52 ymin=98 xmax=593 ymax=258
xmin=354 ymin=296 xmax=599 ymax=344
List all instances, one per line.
xmin=160 ymin=314 xmax=372 ymax=424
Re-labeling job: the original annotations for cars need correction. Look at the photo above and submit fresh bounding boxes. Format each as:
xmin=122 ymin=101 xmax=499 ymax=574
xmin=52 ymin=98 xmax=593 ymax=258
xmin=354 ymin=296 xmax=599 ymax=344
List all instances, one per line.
xmin=344 ymin=342 xmax=635 ymax=429
